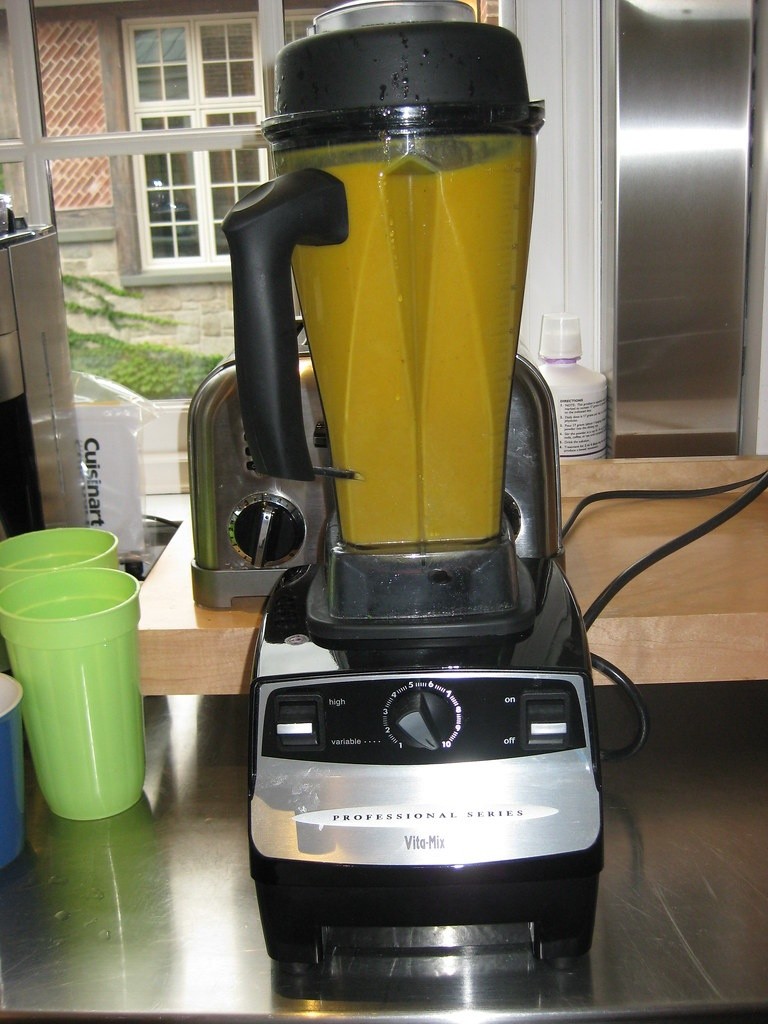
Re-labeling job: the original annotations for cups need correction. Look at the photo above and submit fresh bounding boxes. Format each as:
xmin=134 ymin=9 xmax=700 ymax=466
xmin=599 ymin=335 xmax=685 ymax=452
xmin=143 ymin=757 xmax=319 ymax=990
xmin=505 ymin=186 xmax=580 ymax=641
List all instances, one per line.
xmin=0 ymin=529 xmax=120 ymax=588
xmin=0 ymin=568 xmax=148 ymax=819
xmin=0 ymin=673 xmax=27 ymax=867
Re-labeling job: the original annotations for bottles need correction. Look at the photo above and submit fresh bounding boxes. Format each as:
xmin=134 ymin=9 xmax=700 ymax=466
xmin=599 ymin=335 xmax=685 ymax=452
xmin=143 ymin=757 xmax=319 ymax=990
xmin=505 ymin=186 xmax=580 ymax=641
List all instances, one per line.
xmin=534 ymin=312 xmax=609 ymax=462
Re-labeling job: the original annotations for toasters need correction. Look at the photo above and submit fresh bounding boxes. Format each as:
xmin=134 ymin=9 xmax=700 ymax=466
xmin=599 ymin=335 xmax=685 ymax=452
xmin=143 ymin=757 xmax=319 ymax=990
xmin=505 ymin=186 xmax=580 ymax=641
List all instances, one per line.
xmin=184 ymin=353 xmax=563 ymax=606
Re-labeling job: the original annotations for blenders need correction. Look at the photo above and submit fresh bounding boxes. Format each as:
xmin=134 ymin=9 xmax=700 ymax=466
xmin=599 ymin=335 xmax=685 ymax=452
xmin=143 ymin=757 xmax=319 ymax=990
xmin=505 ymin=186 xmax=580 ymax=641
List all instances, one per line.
xmin=221 ymin=1 xmax=605 ymax=966
